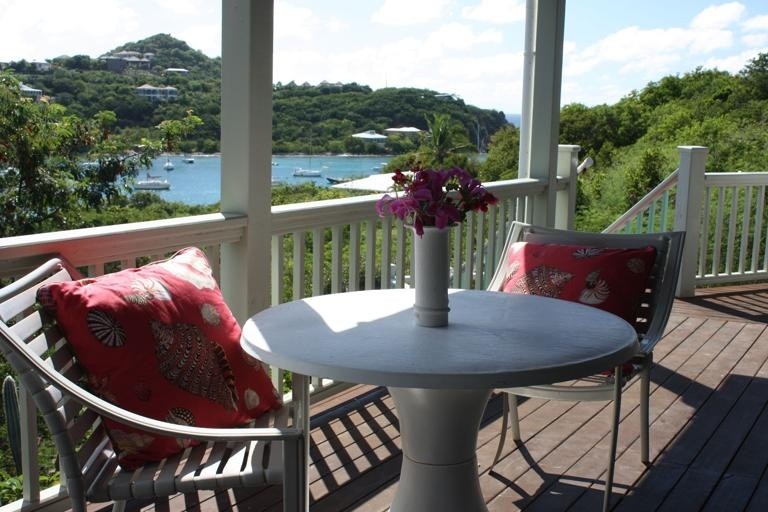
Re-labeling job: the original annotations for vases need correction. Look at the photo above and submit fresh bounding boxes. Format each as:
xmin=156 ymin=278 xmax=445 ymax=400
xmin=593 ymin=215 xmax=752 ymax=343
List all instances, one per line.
xmin=403 ymin=225 xmax=455 ymax=327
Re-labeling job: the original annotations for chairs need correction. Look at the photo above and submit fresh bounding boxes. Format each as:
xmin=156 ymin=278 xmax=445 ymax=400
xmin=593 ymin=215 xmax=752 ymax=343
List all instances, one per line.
xmin=487 ymin=220 xmax=686 ymax=512
xmin=0 ymin=256 xmax=308 ymax=512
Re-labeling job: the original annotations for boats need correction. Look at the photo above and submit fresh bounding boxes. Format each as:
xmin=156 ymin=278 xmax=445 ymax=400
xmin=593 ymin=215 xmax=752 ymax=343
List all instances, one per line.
xmin=164 ymin=160 xmax=175 ymax=171
xmin=81 ymin=157 xmax=123 ymax=167
xmin=135 ymin=173 xmax=171 ymax=190
xmin=327 ymin=175 xmax=353 ymax=185
xmin=181 ymin=155 xmax=195 ymax=164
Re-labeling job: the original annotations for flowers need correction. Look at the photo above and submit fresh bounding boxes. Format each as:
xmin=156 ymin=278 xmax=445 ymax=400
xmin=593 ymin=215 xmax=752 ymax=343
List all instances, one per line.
xmin=376 ymin=166 xmax=500 ymax=239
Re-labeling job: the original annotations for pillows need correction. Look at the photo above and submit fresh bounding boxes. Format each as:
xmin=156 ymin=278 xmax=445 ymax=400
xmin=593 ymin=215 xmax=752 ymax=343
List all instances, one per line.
xmin=36 ymin=246 xmax=284 ymax=474
xmin=502 ymin=242 xmax=657 ymax=375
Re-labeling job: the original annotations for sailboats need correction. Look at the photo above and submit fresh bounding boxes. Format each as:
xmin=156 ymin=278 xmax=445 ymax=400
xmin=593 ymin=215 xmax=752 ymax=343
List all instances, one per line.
xmin=292 ymin=127 xmax=323 ymax=180
